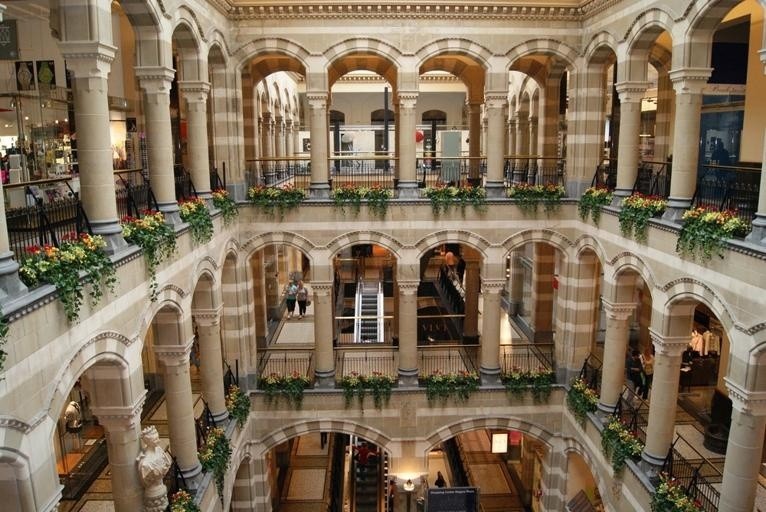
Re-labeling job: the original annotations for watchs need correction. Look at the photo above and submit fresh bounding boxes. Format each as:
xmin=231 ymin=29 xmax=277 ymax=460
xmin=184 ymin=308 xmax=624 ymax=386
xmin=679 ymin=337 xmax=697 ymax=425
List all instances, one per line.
xmin=17 ymin=63 xmax=32 ymax=91
xmin=37 ymin=62 xmax=53 ymax=91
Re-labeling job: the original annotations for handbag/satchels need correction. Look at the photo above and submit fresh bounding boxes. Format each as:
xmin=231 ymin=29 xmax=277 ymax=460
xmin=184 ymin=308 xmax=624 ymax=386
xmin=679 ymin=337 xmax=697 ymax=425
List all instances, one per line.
xmin=305 ymin=300 xmax=310 ymax=306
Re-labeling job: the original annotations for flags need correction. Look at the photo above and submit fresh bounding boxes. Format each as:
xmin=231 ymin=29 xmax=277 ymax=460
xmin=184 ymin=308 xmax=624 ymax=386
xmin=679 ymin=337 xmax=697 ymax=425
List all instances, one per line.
xmin=14 ymin=61 xmax=35 ymax=91
xmin=0 ymin=19 xmax=19 ymax=61
xmin=35 ymin=60 xmax=56 ymax=91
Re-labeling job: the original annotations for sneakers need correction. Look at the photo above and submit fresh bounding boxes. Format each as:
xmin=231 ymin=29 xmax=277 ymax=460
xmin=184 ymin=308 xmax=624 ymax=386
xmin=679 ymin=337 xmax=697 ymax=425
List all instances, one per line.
xmin=287 ymin=313 xmax=304 ymax=319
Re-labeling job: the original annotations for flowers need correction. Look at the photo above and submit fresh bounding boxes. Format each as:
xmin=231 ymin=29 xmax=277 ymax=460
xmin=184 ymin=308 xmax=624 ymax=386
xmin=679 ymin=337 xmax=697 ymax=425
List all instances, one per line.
xmin=513 ymin=179 xmax=564 ymax=195
xmin=432 ymin=183 xmax=478 ymax=195
xmin=607 ymin=413 xmax=644 ymax=457
xmin=200 ymin=424 xmax=223 ymax=461
xmin=343 ymin=369 xmax=391 ymax=387
xmin=505 ymin=365 xmax=553 ymax=380
xmin=224 ymin=383 xmax=240 ymax=411
xmin=334 ymin=180 xmax=388 ymax=195
xmin=681 ymin=205 xmax=743 ymax=234
xmin=18 ymin=231 xmax=103 ymax=258
xmin=622 ymin=191 xmax=667 ymax=212
xmin=426 ymin=368 xmax=477 ymax=386
xmin=265 ymin=369 xmax=313 ymax=387
xmin=658 ymin=479 xmax=701 ymax=511
xmin=249 ymin=182 xmax=296 ymax=198
xmin=212 ymin=187 xmax=230 ymax=202
xmin=121 ymin=207 xmax=166 ymax=232
xmin=178 ymin=193 xmax=203 ymax=216
xmin=583 ymin=186 xmax=609 ymax=197
xmin=169 ymin=487 xmax=192 ymax=511
xmin=572 ymin=377 xmax=599 ymax=405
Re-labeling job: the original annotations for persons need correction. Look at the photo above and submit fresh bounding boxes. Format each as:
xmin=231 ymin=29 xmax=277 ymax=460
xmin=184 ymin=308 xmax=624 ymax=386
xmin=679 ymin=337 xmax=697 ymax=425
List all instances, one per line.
xmin=282 ymin=278 xmax=297 ymax=320
xmin=367 ymin=441 xmax=377 ymax=466
xmin=355 ymin=442 xmax=368 ymax=476
xmin=434 ymin=471 xmax=447 ymax=487
xmin=294 ymin=279 xmax=309 ymax=321
xmin=627 ymin=349 xmax=642 ymax=403
xmin=319 ymin=431 xmax=327 ymax=449
xmin=456 ymin=255 xmax=466 ymax=286
xmin=639 ymin=348 xmax=654 ymax=400
xmin=387 ymin=479 xmax=397 ymax=512
xmin=710 ymin=141 xmax=729 ymax=173
xmin=445 ymin=251 xmax=458 ymax=272
xmin=137 ymin=424 xmax=170 ymax=491
